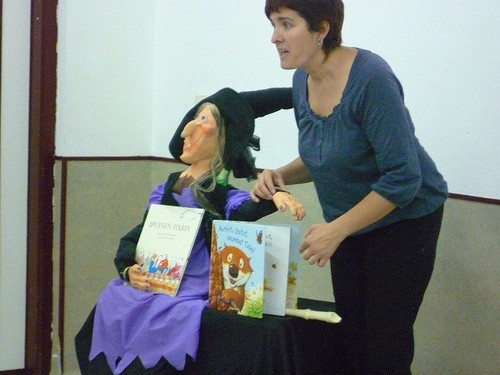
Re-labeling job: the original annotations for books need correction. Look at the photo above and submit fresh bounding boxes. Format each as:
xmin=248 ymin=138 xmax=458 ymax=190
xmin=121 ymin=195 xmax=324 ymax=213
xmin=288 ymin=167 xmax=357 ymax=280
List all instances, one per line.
xmin=133 ymin=203 xmax=205 ymax=297
xmin=210 ymin=219 xmax=301 ymax=318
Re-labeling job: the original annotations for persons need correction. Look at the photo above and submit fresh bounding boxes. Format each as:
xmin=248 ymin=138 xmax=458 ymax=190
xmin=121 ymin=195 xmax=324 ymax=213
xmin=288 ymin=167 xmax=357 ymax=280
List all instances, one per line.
xmin=250 ymin=0 xmax=448 ymax=374
xmin=74 ymin=87 xmax=305 ymax=375
